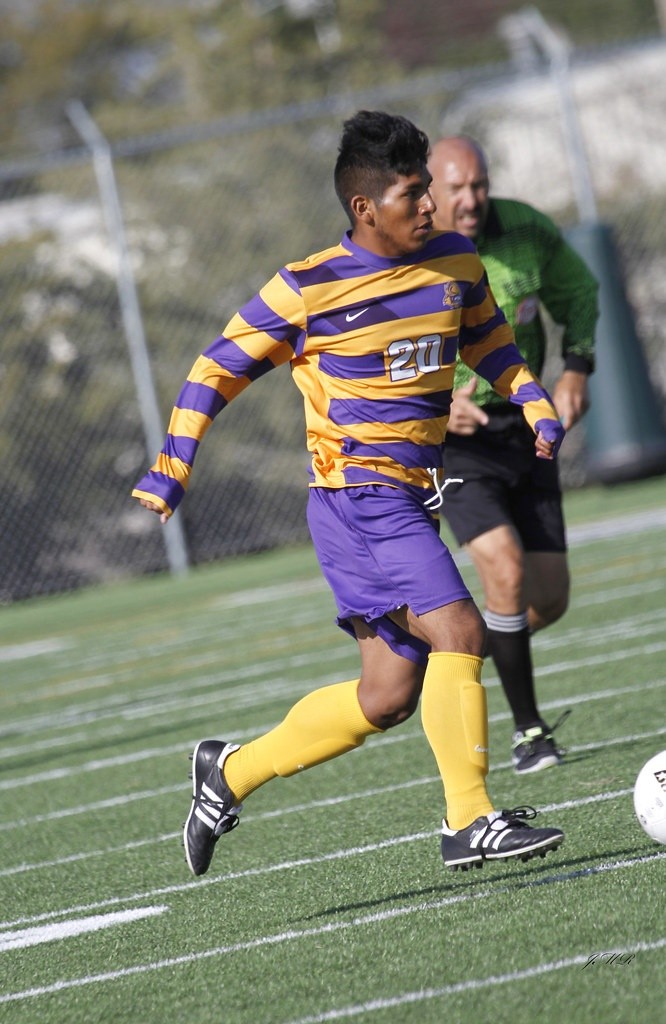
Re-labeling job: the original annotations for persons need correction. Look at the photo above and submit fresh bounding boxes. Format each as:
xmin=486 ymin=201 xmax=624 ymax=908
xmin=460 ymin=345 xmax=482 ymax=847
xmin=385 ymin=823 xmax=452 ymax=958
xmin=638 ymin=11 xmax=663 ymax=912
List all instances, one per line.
xmin=130 ymin=108 xmax=567 ymax=879
xmin=425 ymin=135 xmax=602 ymax=773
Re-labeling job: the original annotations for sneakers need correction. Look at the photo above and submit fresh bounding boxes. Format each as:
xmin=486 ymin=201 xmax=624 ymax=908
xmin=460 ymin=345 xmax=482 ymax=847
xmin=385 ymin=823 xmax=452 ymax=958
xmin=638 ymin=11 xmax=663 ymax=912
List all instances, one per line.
xmin=440 ymin=805 xmax=565 ymax=872
xmin=509 ymin=709 xmax=572 ymax=775
xmin=181 ymin=738 xmax=243 ymax=877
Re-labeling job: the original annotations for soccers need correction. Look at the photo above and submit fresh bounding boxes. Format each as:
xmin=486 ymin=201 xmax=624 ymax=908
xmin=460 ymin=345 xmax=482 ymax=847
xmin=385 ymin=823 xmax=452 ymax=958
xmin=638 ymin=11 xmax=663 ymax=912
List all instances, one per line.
xmin=632 ymin=749 xmax=666 ymax=845
